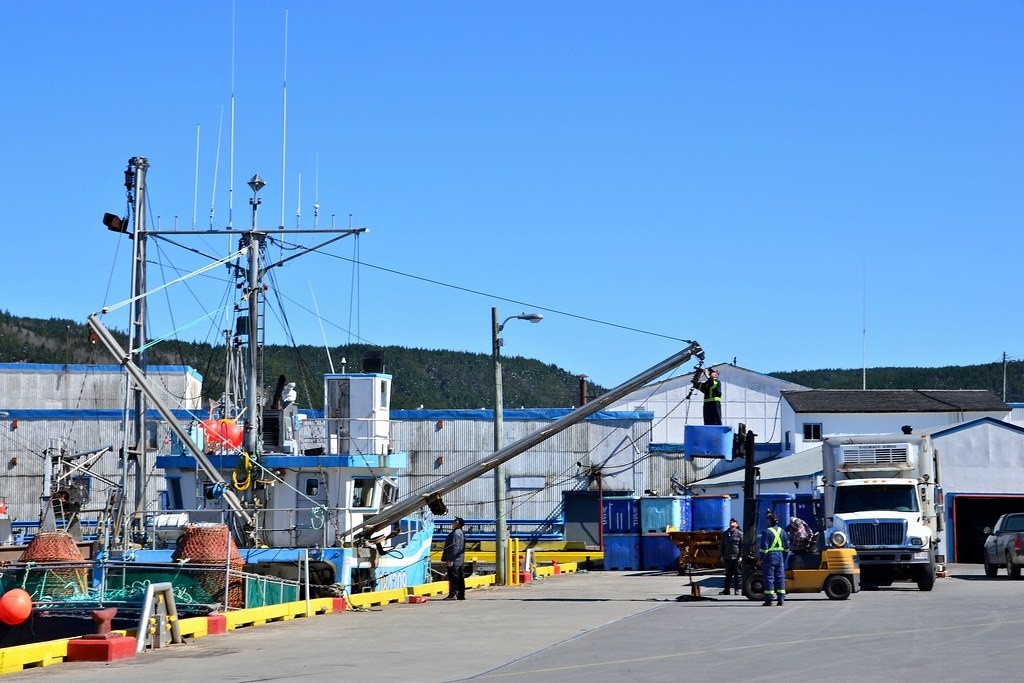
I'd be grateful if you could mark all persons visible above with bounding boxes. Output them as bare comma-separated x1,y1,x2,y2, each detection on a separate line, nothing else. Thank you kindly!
693,367,722,426
760,508,789,607
719,518,744,595
441,516,466,601
785,517,818,569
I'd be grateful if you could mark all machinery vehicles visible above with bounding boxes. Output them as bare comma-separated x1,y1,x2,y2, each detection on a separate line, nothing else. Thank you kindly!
690,422,862,600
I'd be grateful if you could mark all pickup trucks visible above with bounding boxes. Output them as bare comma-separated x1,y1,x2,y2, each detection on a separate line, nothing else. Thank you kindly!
982,512,1024,581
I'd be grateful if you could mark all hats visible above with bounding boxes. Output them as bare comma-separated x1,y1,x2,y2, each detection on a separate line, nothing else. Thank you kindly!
455,516,464,526
731,518,739,524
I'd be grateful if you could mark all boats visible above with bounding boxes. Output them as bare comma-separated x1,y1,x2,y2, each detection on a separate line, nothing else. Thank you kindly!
0,0,439,643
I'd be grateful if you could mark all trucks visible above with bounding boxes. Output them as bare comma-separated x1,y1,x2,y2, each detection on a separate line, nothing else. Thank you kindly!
812,425,946,593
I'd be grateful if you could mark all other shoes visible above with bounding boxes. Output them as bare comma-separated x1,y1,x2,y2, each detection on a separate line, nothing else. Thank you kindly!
776,602,784,606
735,589,740,595
457,595,466,600
762,602,772,606
719,589,730,595
442,595,457,600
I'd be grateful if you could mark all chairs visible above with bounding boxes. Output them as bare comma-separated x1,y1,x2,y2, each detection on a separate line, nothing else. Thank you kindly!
804,531,820,553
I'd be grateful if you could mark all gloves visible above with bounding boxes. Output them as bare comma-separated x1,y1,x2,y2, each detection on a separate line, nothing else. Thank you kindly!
721,556,724,562
737,557,742,565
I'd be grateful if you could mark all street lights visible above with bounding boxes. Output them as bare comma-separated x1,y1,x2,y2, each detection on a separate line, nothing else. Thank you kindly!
490,306,543,586
